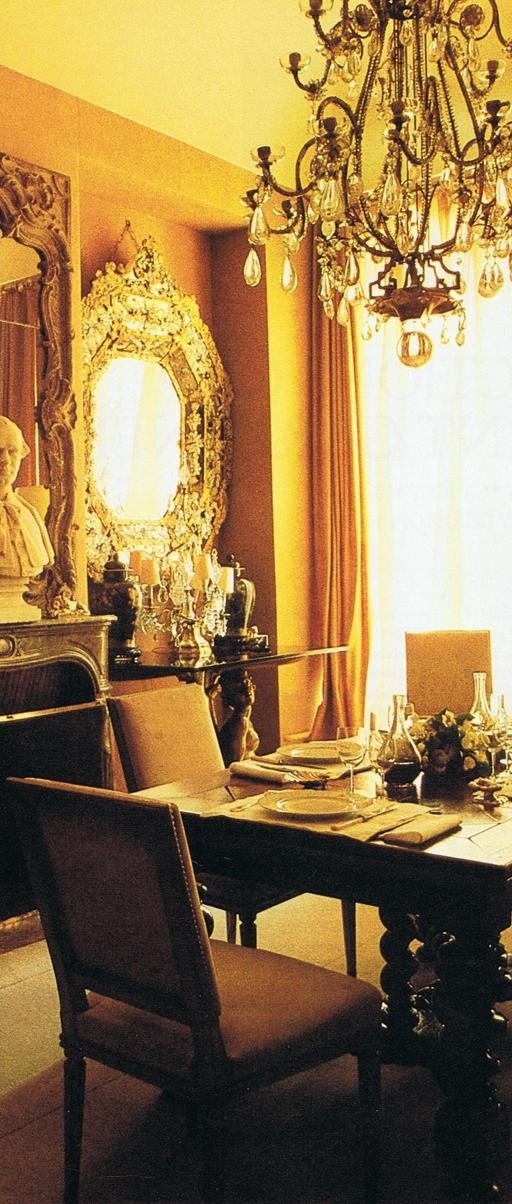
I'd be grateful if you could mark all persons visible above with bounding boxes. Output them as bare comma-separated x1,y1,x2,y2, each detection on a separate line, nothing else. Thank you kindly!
0,414,56,578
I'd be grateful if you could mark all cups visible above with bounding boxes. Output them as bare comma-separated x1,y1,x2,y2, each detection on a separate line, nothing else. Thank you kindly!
335,729,369,798
371,733,396,809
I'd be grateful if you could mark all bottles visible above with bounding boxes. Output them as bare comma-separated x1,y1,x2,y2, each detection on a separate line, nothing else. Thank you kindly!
377,695,421,789
468,670,494,729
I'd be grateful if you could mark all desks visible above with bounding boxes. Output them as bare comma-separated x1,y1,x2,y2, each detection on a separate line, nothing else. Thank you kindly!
101,640,353,766
0,615,121,955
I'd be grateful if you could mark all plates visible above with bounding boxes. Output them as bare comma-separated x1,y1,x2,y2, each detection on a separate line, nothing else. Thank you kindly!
273,738,367,760
256,787,369,817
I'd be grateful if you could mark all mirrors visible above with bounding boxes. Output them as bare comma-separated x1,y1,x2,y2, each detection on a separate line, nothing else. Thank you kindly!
0,170,78,619
81,230,233,646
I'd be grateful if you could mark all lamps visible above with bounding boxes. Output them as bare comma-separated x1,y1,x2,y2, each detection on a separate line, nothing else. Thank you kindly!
240,1,512,367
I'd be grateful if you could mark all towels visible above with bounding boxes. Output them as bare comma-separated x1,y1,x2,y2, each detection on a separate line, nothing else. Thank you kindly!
383,812,462,846
230,762,295,784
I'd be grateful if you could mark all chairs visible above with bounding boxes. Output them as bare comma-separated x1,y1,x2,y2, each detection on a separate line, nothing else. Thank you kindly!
104,683,356,977
3,775,386,1204
404,630,492,720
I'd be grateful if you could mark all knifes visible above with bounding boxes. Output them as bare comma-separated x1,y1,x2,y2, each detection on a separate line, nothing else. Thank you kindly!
326,806,419,830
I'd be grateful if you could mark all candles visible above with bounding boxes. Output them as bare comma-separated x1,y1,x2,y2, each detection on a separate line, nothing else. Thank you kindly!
192,552,212,579
138,558,161,584
128,550,145,575
218,567,234,593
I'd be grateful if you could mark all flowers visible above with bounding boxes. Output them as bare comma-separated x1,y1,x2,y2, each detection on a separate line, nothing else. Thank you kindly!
409,706,492,779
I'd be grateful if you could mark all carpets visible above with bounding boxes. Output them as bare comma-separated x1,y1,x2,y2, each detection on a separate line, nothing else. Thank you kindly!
0,893,511,1203
